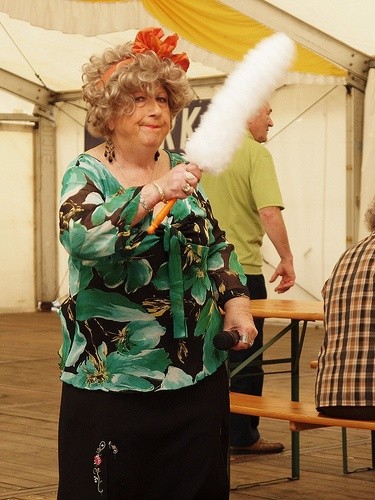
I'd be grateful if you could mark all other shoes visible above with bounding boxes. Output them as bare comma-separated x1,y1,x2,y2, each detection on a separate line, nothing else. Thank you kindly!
230,437,283,455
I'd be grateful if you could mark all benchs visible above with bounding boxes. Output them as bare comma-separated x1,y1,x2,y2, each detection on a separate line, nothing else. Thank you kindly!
230,393,375,431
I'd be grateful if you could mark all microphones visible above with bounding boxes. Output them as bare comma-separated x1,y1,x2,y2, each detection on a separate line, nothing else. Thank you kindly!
213,330,240,350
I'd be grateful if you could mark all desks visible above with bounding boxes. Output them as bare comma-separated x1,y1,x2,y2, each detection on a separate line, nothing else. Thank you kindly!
248,298,325,483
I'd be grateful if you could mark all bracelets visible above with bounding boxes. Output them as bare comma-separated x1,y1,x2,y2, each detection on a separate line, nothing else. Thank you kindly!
152,182,166,203
139,196,154,213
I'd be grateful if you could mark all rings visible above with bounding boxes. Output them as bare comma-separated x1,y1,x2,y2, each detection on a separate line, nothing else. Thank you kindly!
240,335,248,343
184,172,195,180
249,342,253,348
182,182,190,192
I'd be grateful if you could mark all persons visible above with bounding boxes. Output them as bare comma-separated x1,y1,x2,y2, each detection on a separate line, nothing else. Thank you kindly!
314,198,375,421
200,100,296,455
57,27,258,500
